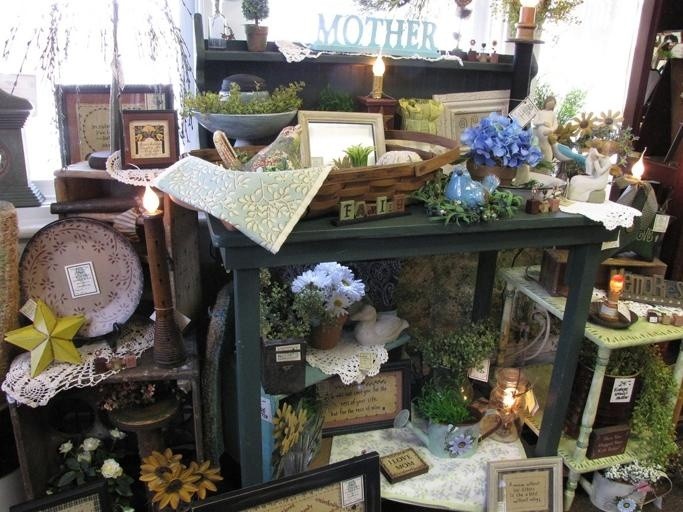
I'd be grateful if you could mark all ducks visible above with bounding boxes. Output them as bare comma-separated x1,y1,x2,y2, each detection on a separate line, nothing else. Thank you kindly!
349,305,410,346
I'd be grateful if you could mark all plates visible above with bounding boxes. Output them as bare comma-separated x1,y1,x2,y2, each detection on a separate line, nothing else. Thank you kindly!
589,302,638,328
17,216,147,341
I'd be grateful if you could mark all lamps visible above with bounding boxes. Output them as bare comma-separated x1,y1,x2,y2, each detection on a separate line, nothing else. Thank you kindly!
141,182,188,368
631,145,647,182
485,366,531,446
372,49,385,99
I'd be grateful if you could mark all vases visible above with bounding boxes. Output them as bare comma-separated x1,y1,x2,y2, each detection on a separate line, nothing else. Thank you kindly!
309,314,346,350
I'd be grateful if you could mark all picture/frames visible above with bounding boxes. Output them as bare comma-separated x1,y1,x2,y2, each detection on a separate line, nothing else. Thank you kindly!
55,84,174,167
433,89,510,140
122,110,179,169
191,451,381,511
322,359,411,438
9,480,110,512
298,110,386,167
486,456,563,512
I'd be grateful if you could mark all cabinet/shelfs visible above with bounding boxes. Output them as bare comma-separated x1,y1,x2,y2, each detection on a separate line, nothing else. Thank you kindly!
490,264,683,475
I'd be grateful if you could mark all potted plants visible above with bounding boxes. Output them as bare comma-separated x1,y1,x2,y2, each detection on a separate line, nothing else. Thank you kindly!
572,343,680,470
180,81,306,147
408,317,497,461
257,267,307,395
242,0,269,52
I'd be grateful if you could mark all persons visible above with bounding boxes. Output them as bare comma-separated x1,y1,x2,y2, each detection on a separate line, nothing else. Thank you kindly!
532,95,559,164
564,138,619,203
583,139,607,176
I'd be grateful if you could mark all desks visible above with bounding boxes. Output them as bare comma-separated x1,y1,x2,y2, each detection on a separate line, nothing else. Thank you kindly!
203,210,619,489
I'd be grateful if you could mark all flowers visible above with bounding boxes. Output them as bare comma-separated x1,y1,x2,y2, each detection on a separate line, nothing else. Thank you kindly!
57,428,223,511
270,404,306,480
290,262,367,318
